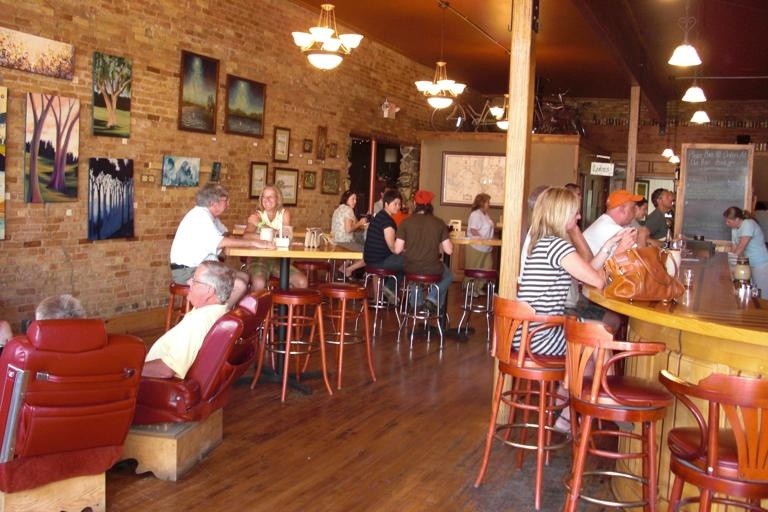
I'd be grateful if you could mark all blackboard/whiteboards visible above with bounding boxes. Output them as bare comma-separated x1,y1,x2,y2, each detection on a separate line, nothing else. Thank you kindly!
673,142,755,246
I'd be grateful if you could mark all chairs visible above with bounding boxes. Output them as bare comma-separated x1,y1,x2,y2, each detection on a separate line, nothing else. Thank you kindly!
109,290,276,486
562,317,672,511
0,317,146,512
472,294,600,511
655,369,768,511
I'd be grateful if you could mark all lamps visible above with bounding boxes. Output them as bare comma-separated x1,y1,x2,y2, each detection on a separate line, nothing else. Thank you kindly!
489,93,509,132
666,3,700,68
291,3,364,71
688,103,711,124
414,0,468,110
681,70,706,102
661,75,681,165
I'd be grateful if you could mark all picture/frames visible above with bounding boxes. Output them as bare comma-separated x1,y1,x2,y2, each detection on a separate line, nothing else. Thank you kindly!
248,161,268,199
273,166,299,207
271,124,290,164
176,48,220,134
303,124,340,195
439,151,505,209
223,73,266,138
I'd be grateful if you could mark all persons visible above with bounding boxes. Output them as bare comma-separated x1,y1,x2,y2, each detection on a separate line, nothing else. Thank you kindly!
241,185,309,292
722,206,768,301
141,259,237,382
0,295,88,353
168,182,249,311
511,183,676,438
331,185,455,314
463,192,495,297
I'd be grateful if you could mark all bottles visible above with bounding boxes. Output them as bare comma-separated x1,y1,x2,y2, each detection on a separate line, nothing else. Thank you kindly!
733,257,751,282
739,283,753,298
751,141,766,151
593,117,766,129
259,229,273,241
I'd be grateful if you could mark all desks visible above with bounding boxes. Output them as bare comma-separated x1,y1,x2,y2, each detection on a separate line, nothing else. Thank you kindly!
220,236,365,396
407,230,503,345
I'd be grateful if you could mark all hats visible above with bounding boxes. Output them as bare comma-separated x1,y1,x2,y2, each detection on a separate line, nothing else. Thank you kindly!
414,190,436,204
606,190,643,208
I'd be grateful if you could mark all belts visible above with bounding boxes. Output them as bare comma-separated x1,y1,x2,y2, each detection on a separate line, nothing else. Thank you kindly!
170,263,188,270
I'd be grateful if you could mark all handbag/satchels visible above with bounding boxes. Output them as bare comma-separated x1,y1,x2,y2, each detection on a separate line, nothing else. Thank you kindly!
604,247,685,301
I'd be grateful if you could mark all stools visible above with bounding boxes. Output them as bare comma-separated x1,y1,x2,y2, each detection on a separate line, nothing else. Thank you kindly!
268,275,302,386
302,282,378,389
165,282,193,332
354,267,400,337
457,269,495,352
249,288,334,401
291,259,335,336
396,271,444,352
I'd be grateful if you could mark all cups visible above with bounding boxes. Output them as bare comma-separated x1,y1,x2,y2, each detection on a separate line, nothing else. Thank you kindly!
669,240,678,250
278,226,294,243
677,239,685,250
446,224,453,233
682,268,694,289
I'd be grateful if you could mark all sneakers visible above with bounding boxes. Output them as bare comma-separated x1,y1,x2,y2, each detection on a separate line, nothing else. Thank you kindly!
335,272,485,312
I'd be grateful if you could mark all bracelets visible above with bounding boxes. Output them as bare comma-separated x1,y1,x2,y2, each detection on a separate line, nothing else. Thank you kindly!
257,221,265,230
